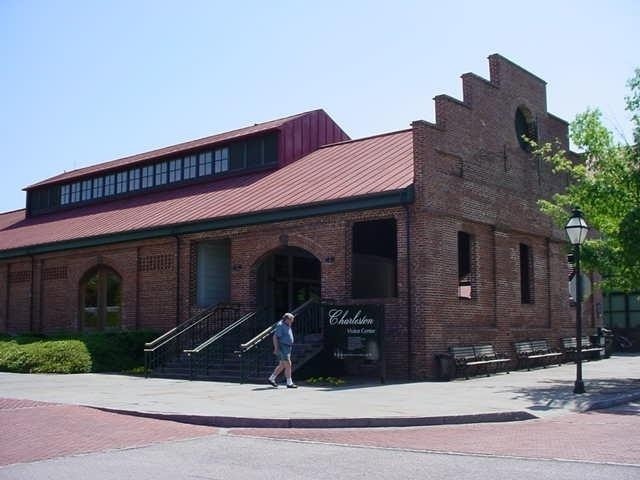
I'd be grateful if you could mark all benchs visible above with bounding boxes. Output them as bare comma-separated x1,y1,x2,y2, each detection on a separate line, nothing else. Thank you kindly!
434,341,513,381
559,335,607,361
510,338,564,371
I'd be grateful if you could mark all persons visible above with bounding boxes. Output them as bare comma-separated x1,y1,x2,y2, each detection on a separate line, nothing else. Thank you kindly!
267,312,298,388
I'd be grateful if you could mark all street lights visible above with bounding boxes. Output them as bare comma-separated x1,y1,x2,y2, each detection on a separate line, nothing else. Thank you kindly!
565,206,591,394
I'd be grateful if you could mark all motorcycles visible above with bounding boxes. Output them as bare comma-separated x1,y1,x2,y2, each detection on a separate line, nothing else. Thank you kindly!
606,322,633,357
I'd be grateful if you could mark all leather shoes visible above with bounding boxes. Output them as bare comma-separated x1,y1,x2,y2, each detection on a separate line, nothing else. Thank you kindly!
267,378,278,387
287,384,298,388
